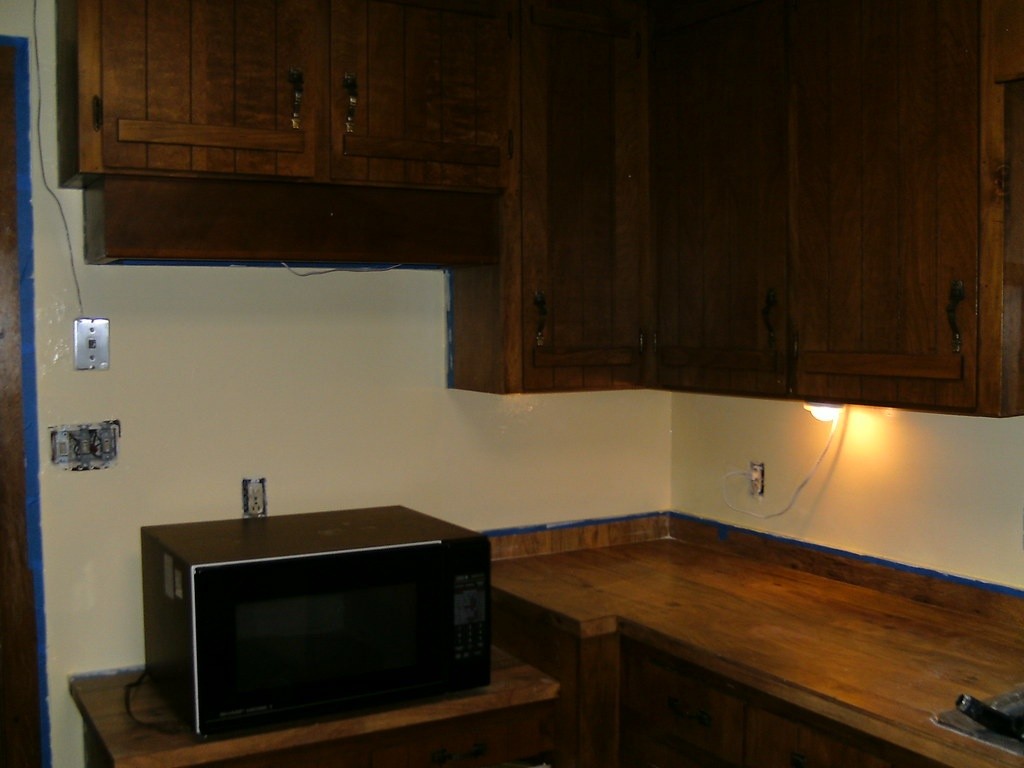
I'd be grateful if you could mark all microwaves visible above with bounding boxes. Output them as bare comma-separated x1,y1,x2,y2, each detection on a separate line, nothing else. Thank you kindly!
140,504,495,739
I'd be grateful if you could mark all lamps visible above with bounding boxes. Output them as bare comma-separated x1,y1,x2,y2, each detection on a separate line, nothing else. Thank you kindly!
800,402,846,423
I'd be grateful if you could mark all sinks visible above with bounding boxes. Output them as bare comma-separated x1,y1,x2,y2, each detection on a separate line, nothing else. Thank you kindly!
927,686,1024,760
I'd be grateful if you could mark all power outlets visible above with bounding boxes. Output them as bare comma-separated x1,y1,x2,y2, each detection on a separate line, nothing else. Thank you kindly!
750,459,766,497
242,476,267,519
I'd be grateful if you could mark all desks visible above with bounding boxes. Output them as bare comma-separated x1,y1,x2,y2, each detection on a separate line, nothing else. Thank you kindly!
66,642,554,768
55,1,514,199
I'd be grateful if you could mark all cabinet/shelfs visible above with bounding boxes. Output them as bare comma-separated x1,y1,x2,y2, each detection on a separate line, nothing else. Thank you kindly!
650,1,1024,417
447,0,644,393
617,620,921,767
